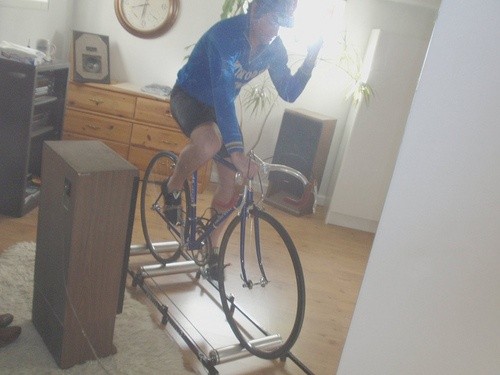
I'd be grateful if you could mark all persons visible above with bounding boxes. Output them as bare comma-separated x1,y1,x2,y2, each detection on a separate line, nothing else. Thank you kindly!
161,0,324,281
0,313,21,349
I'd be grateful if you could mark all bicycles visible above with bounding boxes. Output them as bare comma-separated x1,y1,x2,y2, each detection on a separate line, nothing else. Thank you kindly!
140,149,312,361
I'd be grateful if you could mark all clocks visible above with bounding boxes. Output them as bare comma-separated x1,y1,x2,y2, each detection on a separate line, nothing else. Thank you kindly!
114,0,180,39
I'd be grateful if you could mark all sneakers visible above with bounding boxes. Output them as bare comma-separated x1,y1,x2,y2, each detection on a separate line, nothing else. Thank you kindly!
161,178,183,226
207,254,220,280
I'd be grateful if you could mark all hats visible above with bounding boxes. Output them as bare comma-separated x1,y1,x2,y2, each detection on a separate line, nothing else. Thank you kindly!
260,0,297,27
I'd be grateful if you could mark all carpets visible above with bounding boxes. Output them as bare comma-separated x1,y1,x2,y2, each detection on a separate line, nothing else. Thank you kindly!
0,240,197,375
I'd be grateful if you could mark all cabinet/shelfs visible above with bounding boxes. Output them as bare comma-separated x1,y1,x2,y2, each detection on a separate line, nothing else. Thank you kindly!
0,53,71,219
61,77,215,194
29,139,140,370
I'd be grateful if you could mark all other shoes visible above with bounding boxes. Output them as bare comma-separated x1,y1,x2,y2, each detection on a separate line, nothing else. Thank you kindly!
0,325,21,347
0,313,14,327
